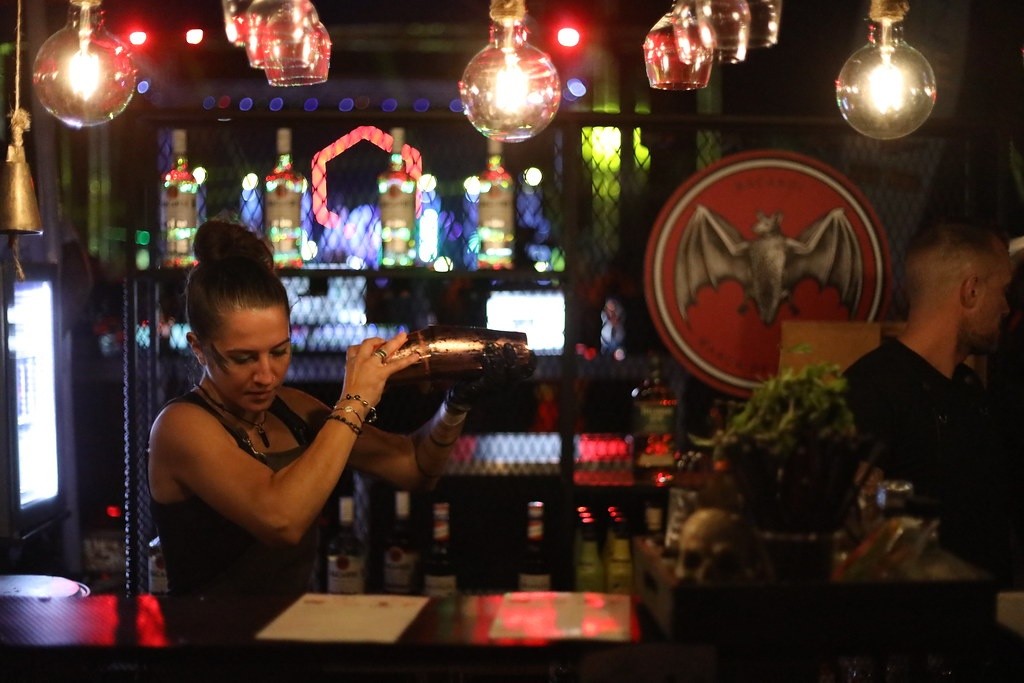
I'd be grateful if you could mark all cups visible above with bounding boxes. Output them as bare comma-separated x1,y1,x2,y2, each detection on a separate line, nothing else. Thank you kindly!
224,0,330,87
642,0,781,89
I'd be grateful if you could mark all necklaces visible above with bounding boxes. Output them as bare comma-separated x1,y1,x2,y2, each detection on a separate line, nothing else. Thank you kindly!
197,384,270,447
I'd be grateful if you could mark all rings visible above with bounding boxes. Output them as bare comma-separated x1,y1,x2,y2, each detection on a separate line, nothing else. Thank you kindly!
374,349,388,363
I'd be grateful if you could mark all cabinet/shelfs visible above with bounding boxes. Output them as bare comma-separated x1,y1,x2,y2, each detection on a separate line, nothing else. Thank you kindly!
77,97,959,596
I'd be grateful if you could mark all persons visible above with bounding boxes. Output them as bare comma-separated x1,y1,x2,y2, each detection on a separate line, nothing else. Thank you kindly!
585,294,642,361
777,221,1024,592
144,219,538,598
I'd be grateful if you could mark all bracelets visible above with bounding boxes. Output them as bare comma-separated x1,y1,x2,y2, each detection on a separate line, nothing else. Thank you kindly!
438,402,465,426
426,421,457,447
325,394,378,435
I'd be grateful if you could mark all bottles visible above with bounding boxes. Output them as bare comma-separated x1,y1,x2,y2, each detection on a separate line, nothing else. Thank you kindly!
158,129,198,268
376,128,417,268
478,138,514,270
631,354,675,477
265,129,304,267
147,533,170,597
327,487,667,598
